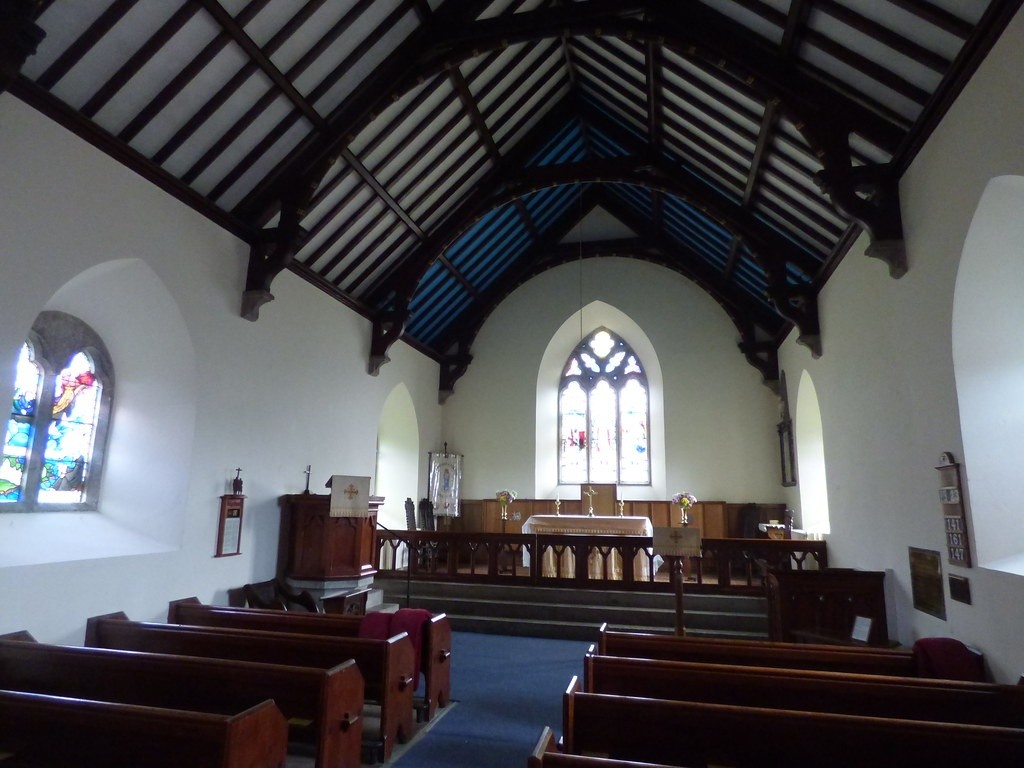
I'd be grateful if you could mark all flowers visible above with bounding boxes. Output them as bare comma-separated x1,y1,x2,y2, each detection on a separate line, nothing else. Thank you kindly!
496,490,517,505
672,492,697,510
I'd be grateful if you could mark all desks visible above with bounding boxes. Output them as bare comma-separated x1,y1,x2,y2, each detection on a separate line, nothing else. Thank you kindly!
522,514,654,581
790,622,899,649
766,568,887,642
701,538,828,594
653,527,700,583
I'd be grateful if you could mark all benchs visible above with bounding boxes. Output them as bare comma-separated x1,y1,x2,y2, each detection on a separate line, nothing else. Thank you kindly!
563,675,1024,766
584,642,1024,729
167,596,453,722
528,725,688,768
0,686,289,768
85,611,419,762
0,629,364,768
598,621,987,684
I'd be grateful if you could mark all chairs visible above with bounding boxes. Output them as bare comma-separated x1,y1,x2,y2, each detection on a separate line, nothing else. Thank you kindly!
744,508,794,586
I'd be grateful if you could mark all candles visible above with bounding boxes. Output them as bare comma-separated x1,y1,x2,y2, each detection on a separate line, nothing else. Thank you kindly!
556,492,559,501
583,486,599,507
621,488,623,503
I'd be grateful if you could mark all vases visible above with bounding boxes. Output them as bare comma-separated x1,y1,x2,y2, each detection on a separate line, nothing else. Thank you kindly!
501,506,507,519
681,508,688,523
